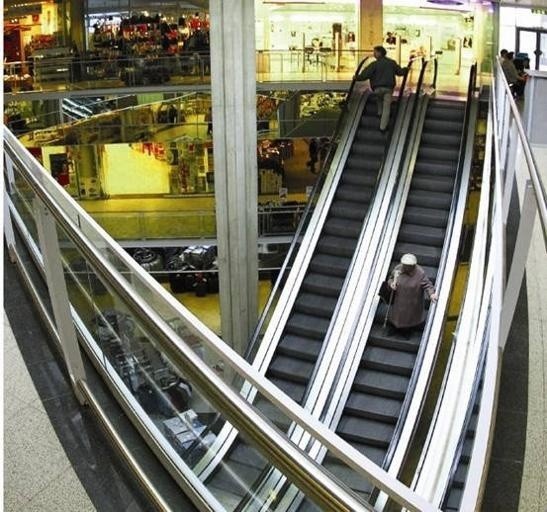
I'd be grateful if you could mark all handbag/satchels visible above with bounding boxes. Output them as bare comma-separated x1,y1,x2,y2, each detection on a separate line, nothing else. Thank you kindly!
378,281,395,305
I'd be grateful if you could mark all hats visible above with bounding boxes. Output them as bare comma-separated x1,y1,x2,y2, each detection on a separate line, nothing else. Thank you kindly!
401,253,417,265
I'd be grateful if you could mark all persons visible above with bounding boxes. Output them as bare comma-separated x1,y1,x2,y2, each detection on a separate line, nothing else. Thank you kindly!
385,253,438,341
353,46,410,133
208,107,212,135
386,32,396,44
316,137,331,169
307,137,319,174
499,49,529,100
